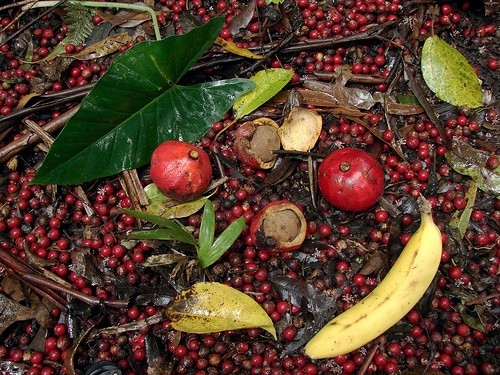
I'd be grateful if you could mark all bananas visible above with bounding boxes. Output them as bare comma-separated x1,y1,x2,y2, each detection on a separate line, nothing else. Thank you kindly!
303,191,443,360
